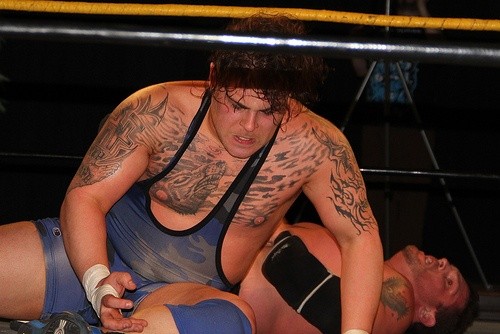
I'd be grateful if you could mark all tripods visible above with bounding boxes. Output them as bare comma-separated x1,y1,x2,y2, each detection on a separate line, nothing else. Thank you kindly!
295,52,493,290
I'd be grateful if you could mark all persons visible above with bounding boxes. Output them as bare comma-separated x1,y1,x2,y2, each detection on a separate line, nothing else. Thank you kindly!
230,217,480,334
351,1,436,124
0,14,384,334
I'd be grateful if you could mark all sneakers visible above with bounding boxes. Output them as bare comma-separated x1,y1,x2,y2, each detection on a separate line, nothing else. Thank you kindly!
10,310,94,334
